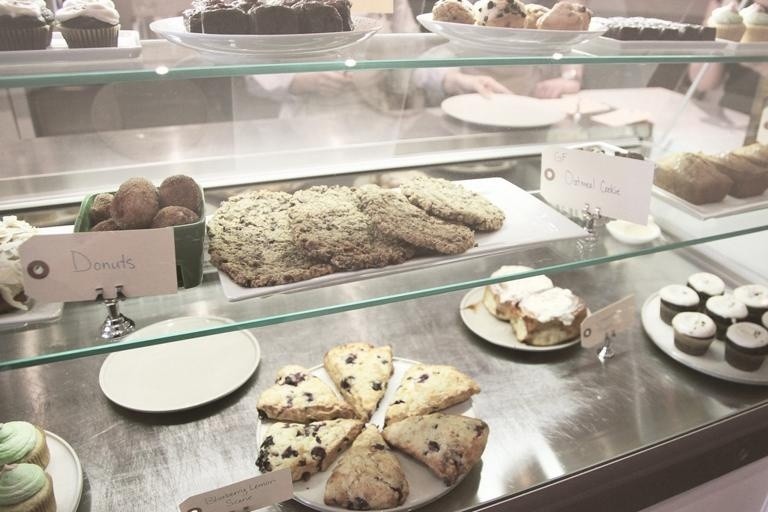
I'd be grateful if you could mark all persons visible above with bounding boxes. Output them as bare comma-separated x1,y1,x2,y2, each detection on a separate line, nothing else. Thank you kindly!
238,0,425,123
689,1,768,128
412,1,591,112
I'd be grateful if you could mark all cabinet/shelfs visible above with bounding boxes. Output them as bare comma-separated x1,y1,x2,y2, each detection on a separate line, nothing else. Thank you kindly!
0,23,768,510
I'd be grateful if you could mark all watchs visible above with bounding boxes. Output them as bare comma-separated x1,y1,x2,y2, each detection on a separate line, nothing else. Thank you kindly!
558,70,585,86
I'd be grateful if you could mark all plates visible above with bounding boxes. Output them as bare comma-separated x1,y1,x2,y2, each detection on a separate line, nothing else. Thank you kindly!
442,93,567,129
98,312,260,413
148,17,381,61
75,191,206,299
415,11,608,51
599,33,726,56
37,426,87,512
459,283,590,353
653,156,766,224
1,294,65,331
2,29,145,73
208,175,588,303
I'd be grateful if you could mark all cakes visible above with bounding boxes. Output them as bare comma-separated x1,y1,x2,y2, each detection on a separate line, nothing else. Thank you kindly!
482,264,587,346
0,421,57,512
0,0,120,51
660,272,768,372
0,215,45,314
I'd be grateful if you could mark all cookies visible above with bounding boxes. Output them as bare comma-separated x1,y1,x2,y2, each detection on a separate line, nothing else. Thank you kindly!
205,176,504,289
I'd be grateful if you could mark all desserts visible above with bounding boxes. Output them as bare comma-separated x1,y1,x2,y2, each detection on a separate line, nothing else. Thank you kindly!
431,1,594,44
593,12,717,41
705,1,768,44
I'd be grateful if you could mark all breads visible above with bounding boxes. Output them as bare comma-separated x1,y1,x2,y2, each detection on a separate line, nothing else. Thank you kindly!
573,143,768,206
182,0,356,35
87,176,202,229
